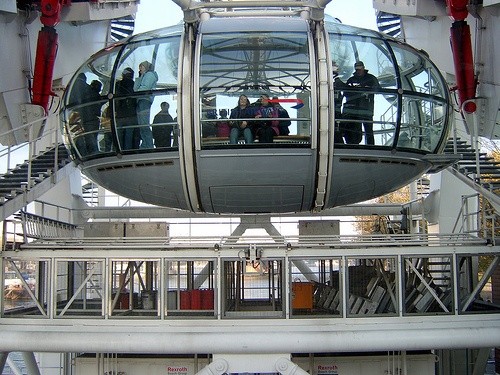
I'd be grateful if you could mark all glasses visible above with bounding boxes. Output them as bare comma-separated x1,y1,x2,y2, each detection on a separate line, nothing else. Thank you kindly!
240,98,247,100
355,67,364,71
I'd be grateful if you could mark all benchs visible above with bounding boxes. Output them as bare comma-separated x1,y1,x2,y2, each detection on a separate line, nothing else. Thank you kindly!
203,136,311,145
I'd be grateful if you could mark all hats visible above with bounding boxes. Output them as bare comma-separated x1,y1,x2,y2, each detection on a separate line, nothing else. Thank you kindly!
354,61,364,67
141,61,154,71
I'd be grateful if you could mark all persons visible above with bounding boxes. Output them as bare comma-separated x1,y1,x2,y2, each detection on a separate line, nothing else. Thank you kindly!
333,62,345,144
228,94,254,144
202,109,230,138
272,97,291,136
69,73,102,157
254,94,279,142
101,67,141,152
152,102,178,147
133,61,158,149
342,62,379,144
